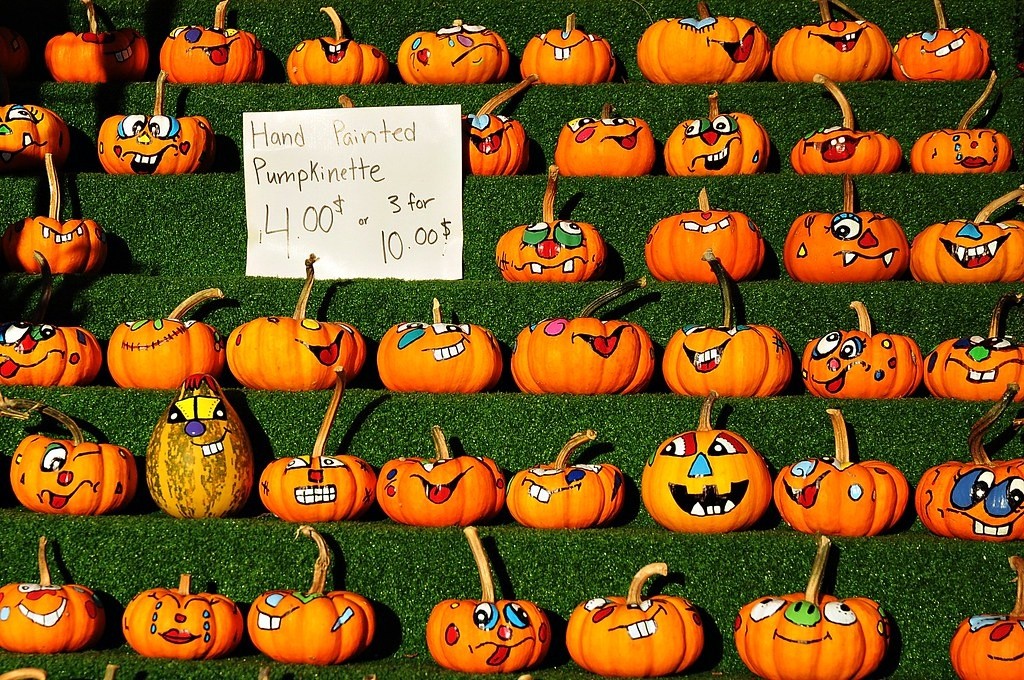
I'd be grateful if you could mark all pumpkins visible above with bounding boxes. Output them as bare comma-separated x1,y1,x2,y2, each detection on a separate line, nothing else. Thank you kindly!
0,0,1024,680
